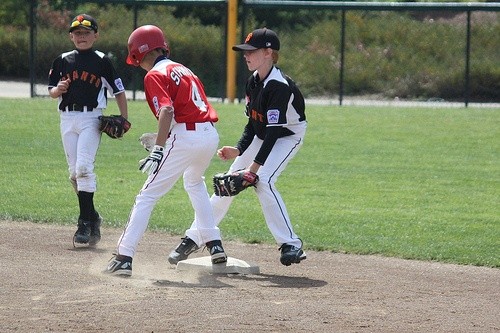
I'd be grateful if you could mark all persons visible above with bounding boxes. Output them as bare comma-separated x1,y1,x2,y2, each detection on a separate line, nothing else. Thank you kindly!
103,24,227,278
47,14,130,247
167,27,308,266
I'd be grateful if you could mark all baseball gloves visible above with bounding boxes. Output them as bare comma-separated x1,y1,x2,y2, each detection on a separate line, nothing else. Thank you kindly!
98,114,132,139
213,168,260,196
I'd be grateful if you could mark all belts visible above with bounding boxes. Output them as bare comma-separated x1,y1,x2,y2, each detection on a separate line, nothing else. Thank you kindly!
62,103,94,113
185,122,195,132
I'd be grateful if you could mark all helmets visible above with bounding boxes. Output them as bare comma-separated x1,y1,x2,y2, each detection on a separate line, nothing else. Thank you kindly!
127,25,170,65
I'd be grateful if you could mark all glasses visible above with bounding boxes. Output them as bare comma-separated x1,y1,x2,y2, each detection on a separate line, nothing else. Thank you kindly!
71,20,97,30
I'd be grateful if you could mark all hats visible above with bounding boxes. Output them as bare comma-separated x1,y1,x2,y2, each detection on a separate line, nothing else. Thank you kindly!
69,14,98,33
232,28,281,51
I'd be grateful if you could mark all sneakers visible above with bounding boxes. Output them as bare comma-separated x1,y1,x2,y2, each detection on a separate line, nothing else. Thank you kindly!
278,240,306,266
168,236,198,265
74,212,102,247
103,252,133,278
205,240,228,264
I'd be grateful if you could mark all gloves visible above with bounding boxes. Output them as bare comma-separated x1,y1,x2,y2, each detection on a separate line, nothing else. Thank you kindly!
138,145,165,176
